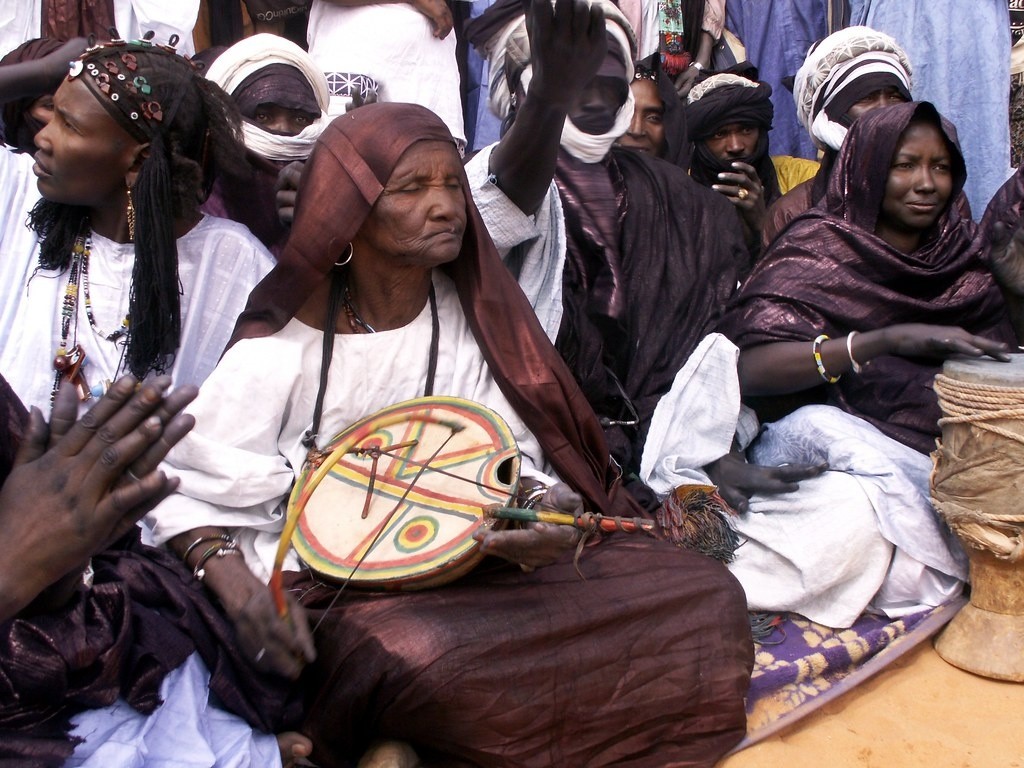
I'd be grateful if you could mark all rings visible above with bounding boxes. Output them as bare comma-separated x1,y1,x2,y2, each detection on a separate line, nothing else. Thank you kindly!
125,469,141,483
737,188,749,199
255,648,265,661
945,339,949,342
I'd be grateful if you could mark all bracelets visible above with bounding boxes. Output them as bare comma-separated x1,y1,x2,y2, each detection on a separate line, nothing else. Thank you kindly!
813,334,841,383
844,329,871,374
182,531,242,579
522,484,549,514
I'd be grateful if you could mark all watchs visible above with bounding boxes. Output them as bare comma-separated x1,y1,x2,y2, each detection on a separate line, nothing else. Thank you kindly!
689,61,704,70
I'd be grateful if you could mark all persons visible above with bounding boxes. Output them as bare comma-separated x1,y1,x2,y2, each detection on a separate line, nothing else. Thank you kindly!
733,100,1023,457
0,0,1010,265
458,1,896,627
137,102,752,768
0,38,280,423
0,369,318,768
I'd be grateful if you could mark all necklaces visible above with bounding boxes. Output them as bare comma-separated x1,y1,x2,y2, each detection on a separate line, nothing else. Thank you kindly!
47,216,134,414
340,284,375,332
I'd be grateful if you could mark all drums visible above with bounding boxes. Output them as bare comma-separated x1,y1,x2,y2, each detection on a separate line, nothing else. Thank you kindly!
932,353,1024,684
284,394,522,591
